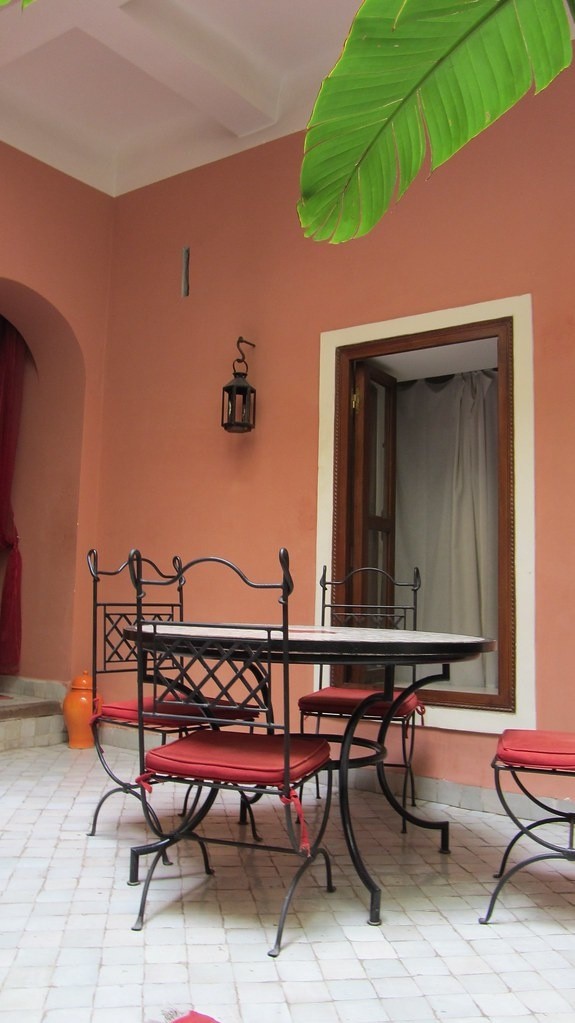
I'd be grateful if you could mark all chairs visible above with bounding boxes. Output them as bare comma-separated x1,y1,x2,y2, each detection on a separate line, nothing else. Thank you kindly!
297,566,425,833
478,729,575,924
85,549,262,874
128,547,338,956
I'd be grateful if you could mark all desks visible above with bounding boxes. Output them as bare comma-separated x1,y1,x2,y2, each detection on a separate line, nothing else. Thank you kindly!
121,623,498,927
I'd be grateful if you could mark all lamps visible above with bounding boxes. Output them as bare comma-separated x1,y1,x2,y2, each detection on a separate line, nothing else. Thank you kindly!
222,337,258,434
